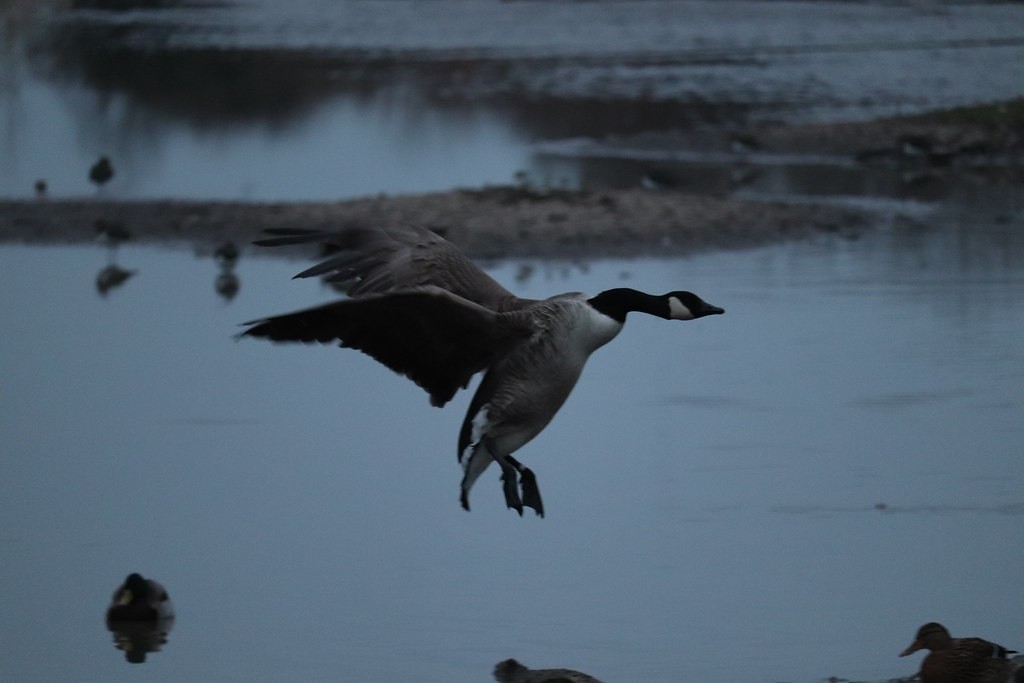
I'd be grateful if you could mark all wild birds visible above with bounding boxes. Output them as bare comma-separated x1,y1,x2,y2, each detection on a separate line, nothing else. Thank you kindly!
900,136,932,158
91,220,130,250
639,171,670,192
97,249,136,299
728,132,762,154
216,268,240,303
106,574,178,629
901,623,1024,683
236,221,724,519
34,180,47,193
493,658,600,683
213,242,236,271
89,158,112,185
